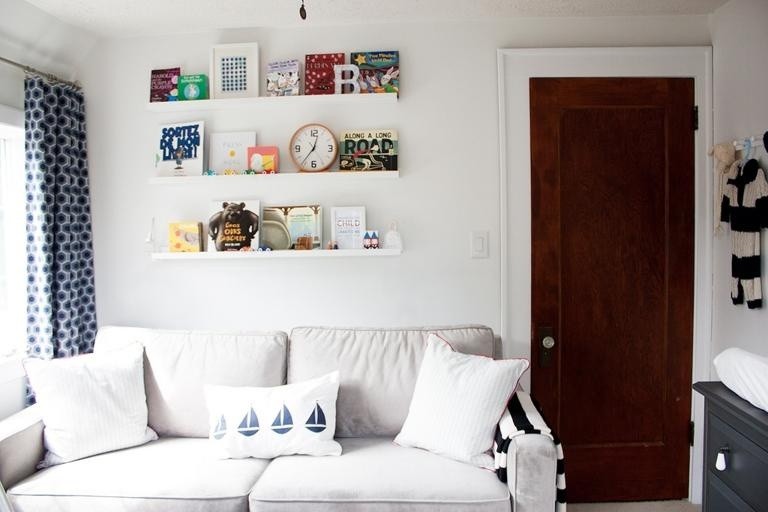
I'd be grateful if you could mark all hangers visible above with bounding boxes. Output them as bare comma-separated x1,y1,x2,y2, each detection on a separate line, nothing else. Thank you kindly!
725,137,765,186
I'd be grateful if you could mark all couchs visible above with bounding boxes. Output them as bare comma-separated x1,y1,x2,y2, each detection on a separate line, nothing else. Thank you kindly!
1,323,559,511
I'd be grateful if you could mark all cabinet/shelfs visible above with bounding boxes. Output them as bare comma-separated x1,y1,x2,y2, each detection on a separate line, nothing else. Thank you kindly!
152,94,401,259
688,375,768,512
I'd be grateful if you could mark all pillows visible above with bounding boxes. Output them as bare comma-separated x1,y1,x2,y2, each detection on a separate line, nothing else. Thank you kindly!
393,334,530,472
22,339,159,470
201,370,343,461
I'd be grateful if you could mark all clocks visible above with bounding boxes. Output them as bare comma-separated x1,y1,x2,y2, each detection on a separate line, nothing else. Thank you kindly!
289,122,339,172
